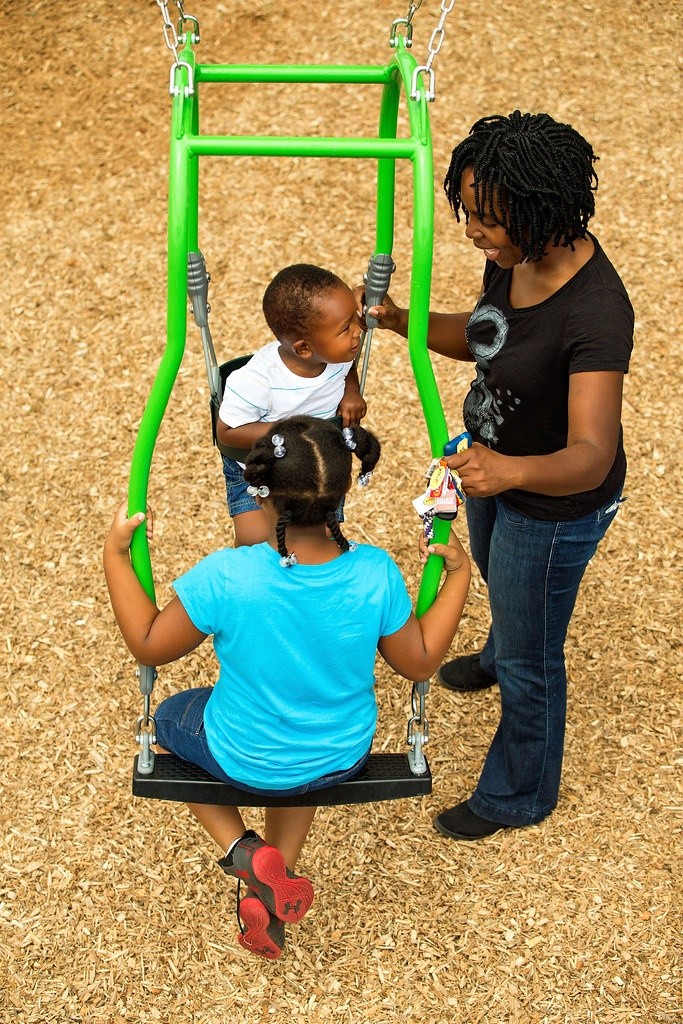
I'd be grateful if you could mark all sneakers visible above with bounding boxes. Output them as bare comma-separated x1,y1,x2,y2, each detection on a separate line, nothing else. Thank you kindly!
218,830,314,924
237,890,285,960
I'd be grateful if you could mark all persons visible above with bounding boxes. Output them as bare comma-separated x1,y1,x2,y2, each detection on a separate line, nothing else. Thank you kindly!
215,263,368,549
103,416,470,958
352,108,636,843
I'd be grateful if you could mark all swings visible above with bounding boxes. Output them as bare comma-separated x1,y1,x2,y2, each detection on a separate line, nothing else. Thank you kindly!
128,0,454,808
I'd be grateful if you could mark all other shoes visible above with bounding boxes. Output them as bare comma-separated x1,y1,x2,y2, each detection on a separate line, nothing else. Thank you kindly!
437,653,498,690
434,801,511,840
